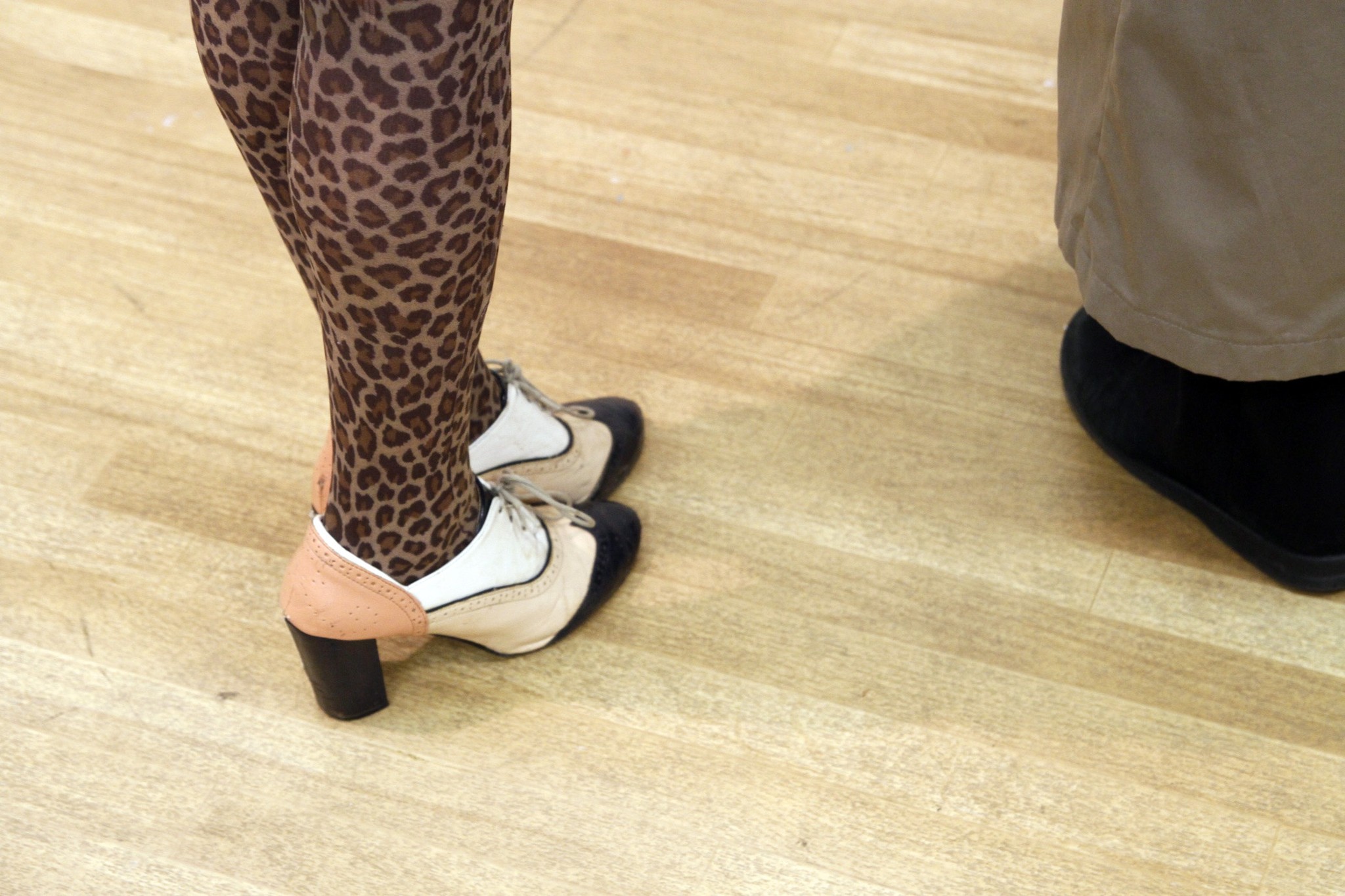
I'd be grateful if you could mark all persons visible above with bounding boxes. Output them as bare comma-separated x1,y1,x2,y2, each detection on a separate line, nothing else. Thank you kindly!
186,0,647,721
1053,0,1345,600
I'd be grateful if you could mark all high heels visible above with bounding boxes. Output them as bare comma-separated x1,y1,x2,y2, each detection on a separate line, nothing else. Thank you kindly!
310,359,644,517
277,472,642,722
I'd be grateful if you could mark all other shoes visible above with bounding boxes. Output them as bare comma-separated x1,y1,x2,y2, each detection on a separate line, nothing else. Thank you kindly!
1060,305,1345,599
